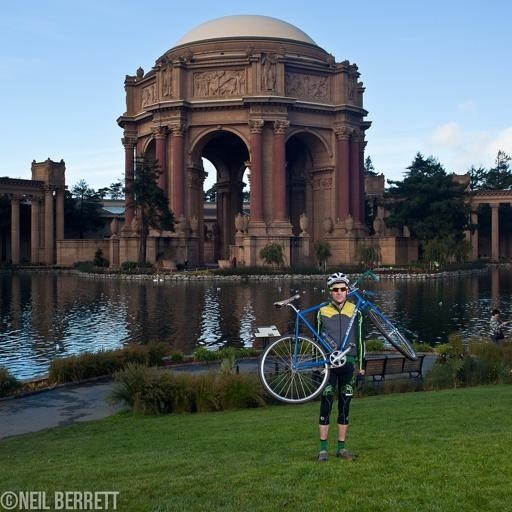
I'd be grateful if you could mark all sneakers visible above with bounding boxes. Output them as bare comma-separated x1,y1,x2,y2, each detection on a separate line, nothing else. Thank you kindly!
335,449,355,460
318,449,329,462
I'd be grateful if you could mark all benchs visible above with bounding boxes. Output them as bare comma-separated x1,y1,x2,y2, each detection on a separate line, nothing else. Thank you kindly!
364,355,425,385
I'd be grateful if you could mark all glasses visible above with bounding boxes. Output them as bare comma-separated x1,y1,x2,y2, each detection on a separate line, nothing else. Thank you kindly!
329,286,348,292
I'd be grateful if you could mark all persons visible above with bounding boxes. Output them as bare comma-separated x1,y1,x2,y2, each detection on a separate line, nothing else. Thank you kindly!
489,308,506,346
371,261,377,269
184,258,189,271
360,260,368,274
319,261,322,271
232,255,237,268
311,271,368,463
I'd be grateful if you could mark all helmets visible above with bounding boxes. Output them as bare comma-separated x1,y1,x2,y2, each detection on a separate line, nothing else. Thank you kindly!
326,271,350,288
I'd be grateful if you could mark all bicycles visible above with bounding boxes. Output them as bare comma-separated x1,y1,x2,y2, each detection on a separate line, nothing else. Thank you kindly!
258,271,417,404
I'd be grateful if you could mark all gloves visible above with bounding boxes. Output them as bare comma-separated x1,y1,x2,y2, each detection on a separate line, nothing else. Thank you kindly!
355,372,364,391
311,369,323,389
